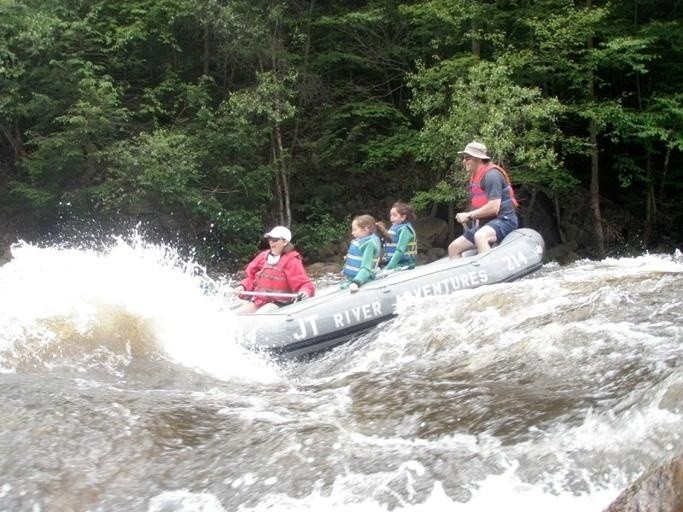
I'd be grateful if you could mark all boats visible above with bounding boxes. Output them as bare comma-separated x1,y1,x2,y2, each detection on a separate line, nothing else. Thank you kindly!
230,226,548,370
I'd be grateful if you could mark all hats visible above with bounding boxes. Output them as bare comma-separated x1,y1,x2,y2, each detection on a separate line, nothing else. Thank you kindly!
457,142,491,160
264,226,292,241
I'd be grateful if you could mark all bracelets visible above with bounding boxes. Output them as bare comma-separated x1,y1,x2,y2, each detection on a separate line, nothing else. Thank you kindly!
466,212,472,220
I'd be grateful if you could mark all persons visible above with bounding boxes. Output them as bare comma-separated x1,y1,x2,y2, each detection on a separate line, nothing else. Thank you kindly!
444,142,519,260
222,225,315,316
377,201,419,277
337,214,393,294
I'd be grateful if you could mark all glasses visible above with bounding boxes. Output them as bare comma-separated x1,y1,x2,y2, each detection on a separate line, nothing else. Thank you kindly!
462,156,470,161
